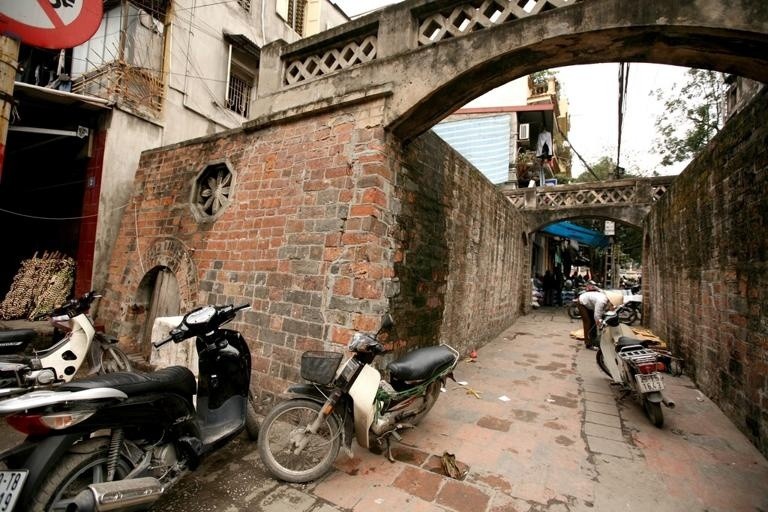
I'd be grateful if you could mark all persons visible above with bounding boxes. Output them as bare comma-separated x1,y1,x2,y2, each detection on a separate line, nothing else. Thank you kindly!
542,269,555,307
576,290,624,351
552,266,566,307
534,273,543,289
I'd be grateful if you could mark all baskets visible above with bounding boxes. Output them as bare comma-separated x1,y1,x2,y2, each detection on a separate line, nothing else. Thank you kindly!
300,346,344,386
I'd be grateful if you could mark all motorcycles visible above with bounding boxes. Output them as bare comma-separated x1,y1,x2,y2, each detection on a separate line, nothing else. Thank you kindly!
256,309,461,480
0,290,133,399
567,272,645,326
594,300,677,427
0,302,259,511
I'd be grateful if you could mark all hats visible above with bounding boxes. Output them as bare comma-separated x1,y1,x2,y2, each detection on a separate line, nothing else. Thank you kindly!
603,290,623,309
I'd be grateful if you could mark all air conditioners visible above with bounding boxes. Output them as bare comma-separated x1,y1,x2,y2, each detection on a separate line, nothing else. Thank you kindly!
519,123,532,148
